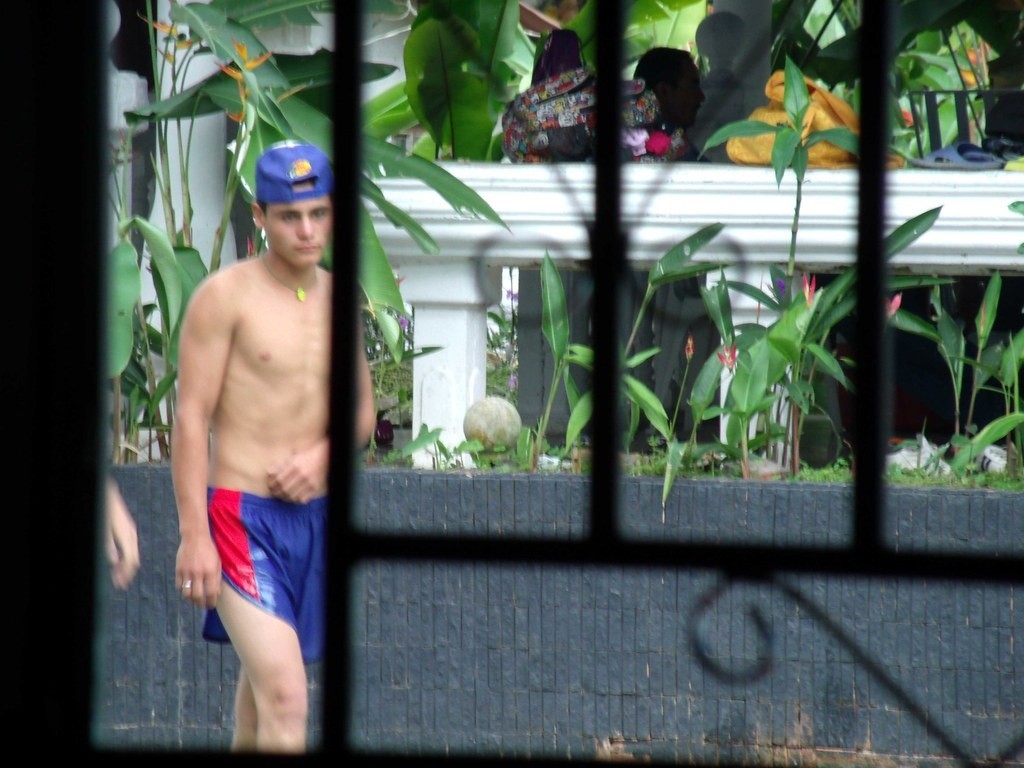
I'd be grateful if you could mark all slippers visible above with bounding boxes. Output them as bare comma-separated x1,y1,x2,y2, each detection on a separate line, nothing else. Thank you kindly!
911,140,1003,170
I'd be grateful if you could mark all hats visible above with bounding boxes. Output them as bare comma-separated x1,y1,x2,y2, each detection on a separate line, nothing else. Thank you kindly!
254,139,334,204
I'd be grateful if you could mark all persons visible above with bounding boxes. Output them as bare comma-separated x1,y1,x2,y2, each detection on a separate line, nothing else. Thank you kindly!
169,140,375,754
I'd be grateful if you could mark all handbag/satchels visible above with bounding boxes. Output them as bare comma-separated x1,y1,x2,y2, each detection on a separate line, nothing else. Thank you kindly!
501,67,686,165
725,71,905,169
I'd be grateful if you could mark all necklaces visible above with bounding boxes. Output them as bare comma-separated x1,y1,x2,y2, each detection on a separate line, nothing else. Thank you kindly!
260,255,315,302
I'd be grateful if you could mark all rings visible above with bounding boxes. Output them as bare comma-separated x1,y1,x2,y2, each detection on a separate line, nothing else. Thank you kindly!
181,580,192,588
